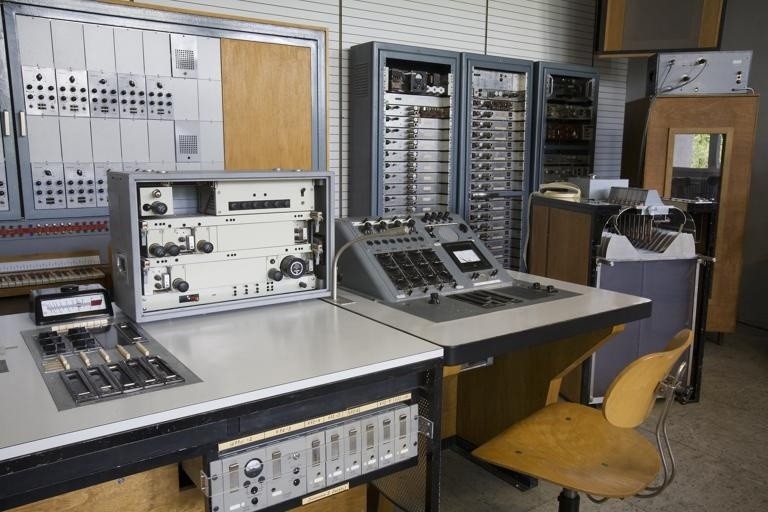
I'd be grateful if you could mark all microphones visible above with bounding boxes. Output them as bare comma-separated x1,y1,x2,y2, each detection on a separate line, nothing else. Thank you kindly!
324,225,410,306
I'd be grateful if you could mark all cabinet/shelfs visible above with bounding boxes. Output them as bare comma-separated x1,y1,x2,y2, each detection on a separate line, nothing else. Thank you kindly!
623,93,760,345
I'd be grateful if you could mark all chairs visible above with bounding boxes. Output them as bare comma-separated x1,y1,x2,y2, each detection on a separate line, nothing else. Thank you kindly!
471,329,697,512
